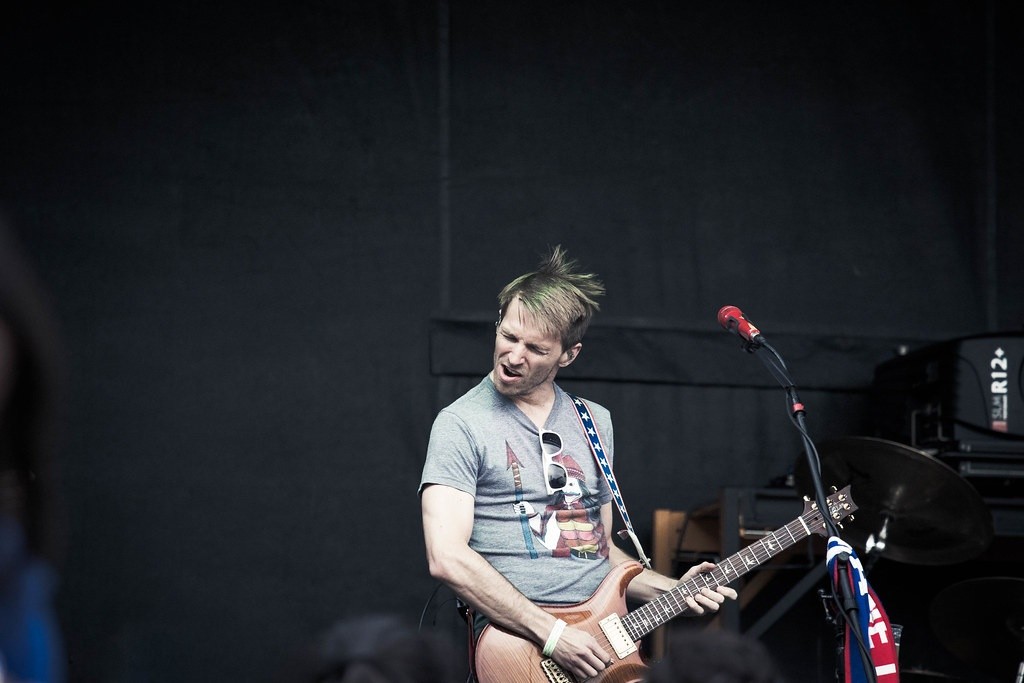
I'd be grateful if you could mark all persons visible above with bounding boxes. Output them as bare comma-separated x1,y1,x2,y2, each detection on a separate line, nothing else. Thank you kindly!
418,245,739,683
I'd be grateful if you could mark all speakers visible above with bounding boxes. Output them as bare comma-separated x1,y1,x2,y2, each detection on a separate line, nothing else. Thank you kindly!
646,484,842,683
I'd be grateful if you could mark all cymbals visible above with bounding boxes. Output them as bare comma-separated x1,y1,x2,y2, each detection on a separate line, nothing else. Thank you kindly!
789,427,998,572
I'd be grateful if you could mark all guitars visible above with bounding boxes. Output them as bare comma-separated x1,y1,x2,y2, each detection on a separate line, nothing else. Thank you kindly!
468,475,871,683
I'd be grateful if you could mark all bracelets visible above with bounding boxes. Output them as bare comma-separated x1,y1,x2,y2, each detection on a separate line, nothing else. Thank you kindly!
541,618,567,656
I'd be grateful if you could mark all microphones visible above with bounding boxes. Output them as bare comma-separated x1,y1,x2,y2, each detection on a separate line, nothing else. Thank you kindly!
717,305,766,346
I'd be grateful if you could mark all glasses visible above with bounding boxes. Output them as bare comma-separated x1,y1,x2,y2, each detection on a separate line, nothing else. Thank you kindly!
539,428,568,496
